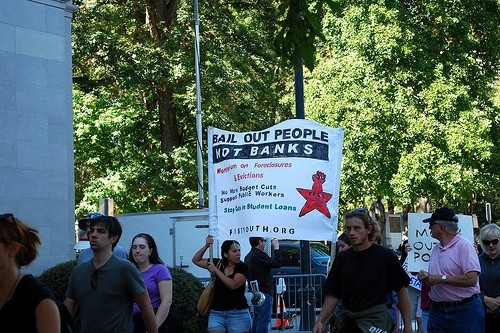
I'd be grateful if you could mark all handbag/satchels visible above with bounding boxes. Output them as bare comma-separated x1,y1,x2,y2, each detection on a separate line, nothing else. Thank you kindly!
197,260,223,315
421,290,431,309
57,302,74,333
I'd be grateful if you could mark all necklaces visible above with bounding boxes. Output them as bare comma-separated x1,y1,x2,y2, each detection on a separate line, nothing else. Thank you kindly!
5,270,21,305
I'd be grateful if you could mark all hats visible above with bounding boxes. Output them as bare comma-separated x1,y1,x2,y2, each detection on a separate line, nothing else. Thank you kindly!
79,213,104,231
423,208,458,223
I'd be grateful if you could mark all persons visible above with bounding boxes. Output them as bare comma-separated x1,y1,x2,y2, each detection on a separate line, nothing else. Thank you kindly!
63,217,158,333
336,223,483,333
244,237,282,333
417,208,484,333
191,234,252,333
477,224,500,333
312,209,413,333
128,233,172,329
0,212,61,333
76,213,128,268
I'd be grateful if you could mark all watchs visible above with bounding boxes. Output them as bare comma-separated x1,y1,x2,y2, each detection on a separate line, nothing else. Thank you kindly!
441,275,447,283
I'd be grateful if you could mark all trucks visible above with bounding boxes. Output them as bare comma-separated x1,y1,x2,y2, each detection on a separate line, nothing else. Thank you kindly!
114,207,332,308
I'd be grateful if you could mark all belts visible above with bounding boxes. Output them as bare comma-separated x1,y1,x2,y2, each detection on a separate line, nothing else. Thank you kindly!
430,292,480,307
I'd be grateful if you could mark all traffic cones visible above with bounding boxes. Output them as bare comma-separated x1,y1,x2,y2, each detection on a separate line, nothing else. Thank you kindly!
271,293,294,330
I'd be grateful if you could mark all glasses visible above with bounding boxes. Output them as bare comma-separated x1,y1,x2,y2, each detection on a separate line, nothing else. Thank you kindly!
358,208,371,223
481,237,499,246
91,270,97,291
258,239,266,243
0,214,20,243
430,221,446,227
